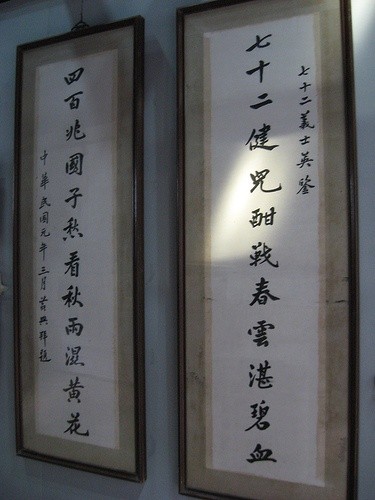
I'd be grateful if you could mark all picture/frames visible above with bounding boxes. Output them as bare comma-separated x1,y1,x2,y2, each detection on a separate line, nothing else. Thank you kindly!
11,15,148,485
175,0,358,500
